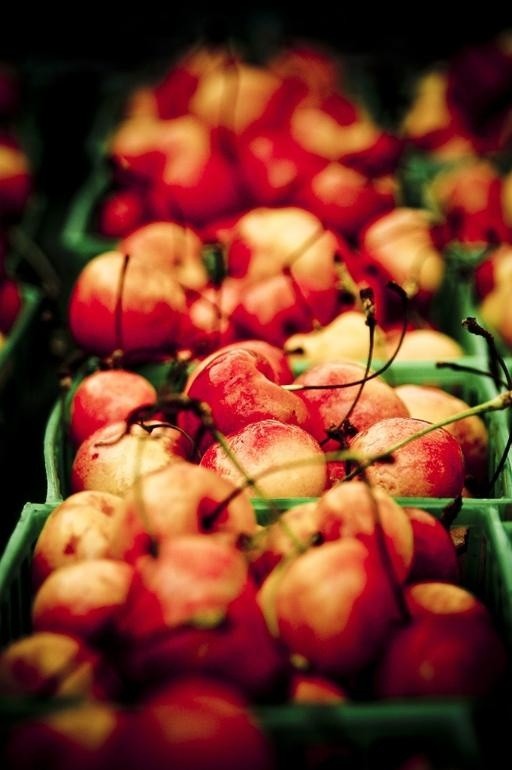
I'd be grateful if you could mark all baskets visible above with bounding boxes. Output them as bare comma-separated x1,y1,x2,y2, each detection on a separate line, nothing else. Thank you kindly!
0,94,512,770
2,41,510,769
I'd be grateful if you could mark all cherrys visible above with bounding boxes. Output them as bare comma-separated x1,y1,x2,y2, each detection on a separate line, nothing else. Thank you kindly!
0,0,512,770
